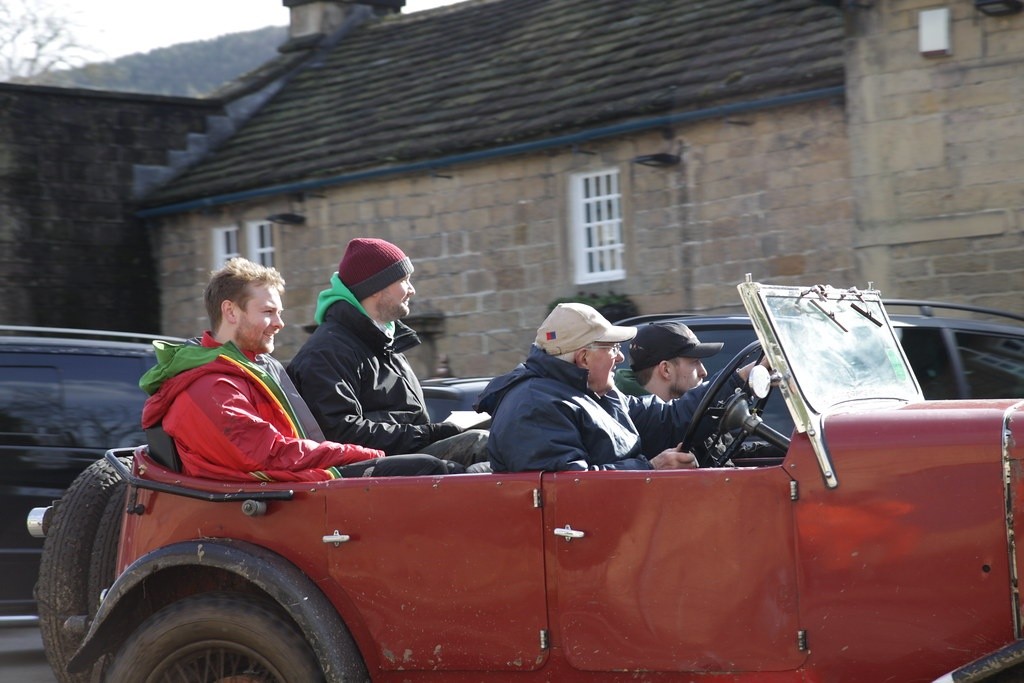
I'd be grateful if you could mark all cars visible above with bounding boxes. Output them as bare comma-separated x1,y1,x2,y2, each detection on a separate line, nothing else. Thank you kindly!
614,315,1023,445
421,376,497,420
22,274,1024,683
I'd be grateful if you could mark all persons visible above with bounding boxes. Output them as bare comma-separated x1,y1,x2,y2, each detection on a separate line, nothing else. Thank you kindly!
612,320,769,468
469,303,772,473
283,237,491,466
138,256,466,483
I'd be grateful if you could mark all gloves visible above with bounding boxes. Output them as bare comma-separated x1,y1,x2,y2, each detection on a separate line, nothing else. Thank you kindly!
425,422,468,444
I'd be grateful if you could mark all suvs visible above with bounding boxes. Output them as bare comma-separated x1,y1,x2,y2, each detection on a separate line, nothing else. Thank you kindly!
0,324,186,627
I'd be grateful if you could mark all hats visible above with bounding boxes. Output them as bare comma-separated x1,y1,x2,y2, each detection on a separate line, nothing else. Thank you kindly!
629,322,725,372
338,238,414,303
536,302,638,356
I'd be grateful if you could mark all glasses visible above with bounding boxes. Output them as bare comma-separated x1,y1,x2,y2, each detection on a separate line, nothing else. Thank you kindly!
585,342,622,360
